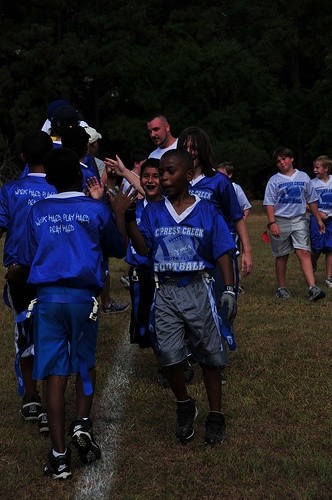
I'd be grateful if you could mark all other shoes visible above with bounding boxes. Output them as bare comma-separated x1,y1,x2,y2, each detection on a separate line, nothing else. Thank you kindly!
325,277,332,288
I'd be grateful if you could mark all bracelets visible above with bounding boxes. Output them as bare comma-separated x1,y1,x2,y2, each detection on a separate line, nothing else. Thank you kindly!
267,222,276,228
224,285,236,293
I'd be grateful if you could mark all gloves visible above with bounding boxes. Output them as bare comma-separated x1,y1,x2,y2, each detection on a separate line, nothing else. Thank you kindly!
220,285,238,322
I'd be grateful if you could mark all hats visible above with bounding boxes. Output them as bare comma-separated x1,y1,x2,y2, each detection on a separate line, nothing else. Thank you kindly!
47,100,80,130
84,127,102,144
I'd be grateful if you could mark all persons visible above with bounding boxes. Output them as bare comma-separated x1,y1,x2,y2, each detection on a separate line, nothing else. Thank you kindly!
125,149,236,444
17,147,128,480
306,156,332,287
263,146,325,302
0,98,253,434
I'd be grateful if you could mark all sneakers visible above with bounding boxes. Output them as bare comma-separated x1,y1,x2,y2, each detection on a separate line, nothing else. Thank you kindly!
276,287,290,298
174,395,199,444
67,417,101,465
19,396,42,421
308,285,326,302
43,447,73,481
36,412,49,434
204,411,226,445
100,299,130,315
